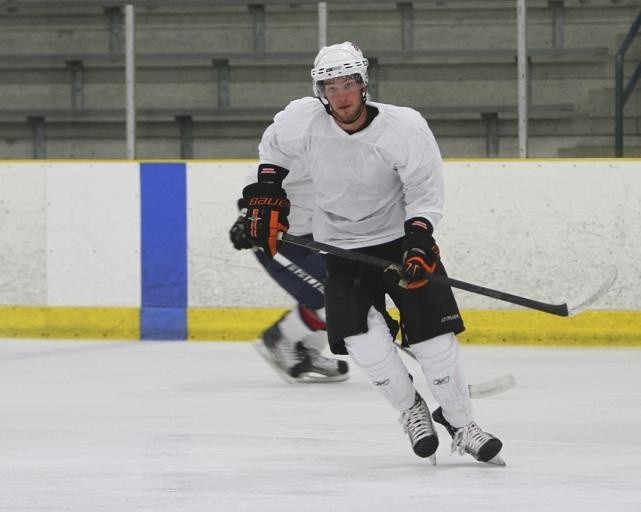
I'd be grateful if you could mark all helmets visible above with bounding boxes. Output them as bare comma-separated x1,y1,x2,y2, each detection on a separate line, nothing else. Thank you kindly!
310,41,370,105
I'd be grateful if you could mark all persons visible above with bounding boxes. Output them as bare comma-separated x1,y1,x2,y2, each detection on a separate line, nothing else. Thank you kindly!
252,231,348,388
229,39,502,463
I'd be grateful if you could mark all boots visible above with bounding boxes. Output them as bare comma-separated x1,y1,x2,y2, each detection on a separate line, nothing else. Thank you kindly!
263,311,349,377
396,372,439,458
432,406,503,462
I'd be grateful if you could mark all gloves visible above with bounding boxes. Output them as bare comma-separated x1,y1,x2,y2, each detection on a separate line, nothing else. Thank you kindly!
400,218,440,290
230,183,292,259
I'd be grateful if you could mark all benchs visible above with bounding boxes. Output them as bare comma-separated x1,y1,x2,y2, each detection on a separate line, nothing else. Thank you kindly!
0,1,640,158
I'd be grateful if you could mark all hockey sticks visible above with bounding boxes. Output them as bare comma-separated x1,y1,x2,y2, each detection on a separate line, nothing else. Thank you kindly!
266,255,515,399
275,230,618,318
